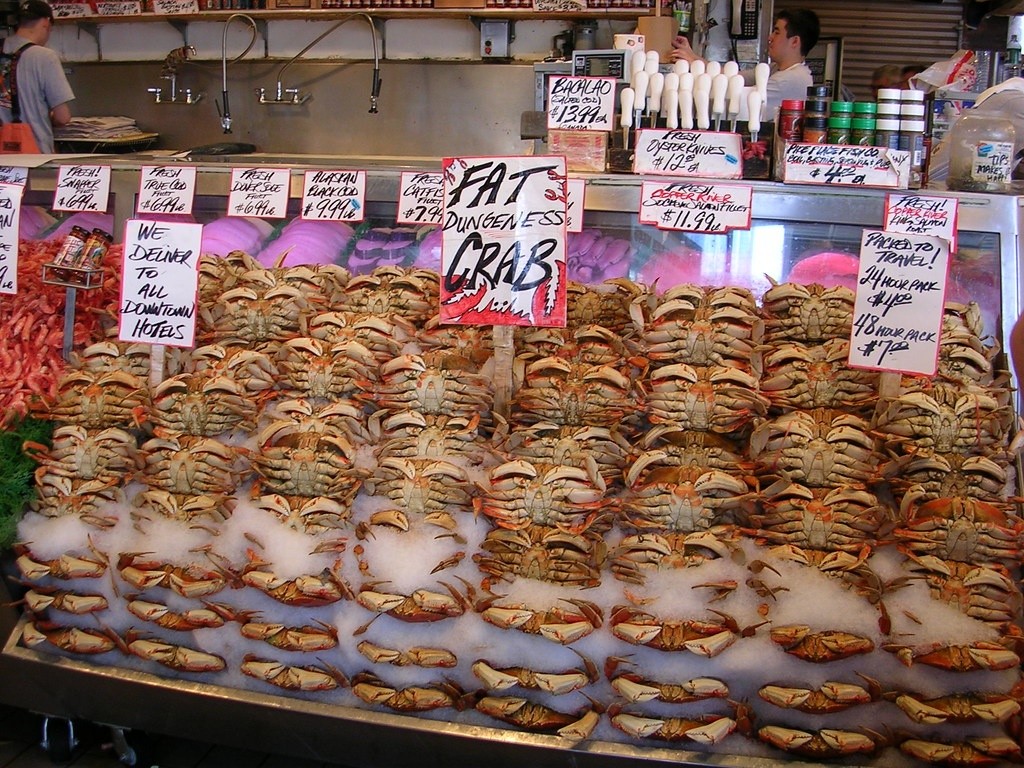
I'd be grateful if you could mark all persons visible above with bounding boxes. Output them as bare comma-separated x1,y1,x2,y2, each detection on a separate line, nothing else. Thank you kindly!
0,0,76,154
667,9,821,125
869,65,932,102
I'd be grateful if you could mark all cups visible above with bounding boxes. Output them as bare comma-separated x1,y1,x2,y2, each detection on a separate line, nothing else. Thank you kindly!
1003,63,1024,80
673,10,691,32
974,50,991,92
993,52,1009,86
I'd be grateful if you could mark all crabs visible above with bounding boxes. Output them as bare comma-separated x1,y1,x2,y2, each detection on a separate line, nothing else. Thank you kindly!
0,250,1024,768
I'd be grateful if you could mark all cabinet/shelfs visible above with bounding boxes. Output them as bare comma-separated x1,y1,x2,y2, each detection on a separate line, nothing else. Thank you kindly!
45,0,695,71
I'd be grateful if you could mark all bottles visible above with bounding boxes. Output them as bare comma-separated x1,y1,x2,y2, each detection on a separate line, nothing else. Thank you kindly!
1007,35,1022,65
947,109,1014,192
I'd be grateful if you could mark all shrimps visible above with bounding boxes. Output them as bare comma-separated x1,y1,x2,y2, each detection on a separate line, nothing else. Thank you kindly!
0,232,126,433
